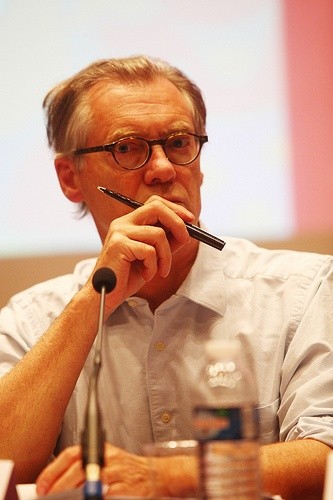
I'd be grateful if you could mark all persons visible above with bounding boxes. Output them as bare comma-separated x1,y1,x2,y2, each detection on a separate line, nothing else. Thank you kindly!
0,56,333,500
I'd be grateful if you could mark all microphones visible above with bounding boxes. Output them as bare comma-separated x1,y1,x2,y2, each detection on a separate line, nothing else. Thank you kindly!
79,267,117,500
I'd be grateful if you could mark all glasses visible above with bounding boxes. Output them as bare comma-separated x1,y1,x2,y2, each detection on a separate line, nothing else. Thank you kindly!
72,132,208,170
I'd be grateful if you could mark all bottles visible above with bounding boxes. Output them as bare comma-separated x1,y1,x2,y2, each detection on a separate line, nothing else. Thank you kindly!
192,338,261,500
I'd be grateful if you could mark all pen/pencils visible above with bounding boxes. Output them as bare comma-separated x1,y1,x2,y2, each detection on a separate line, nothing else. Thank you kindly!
96,185,227,252
